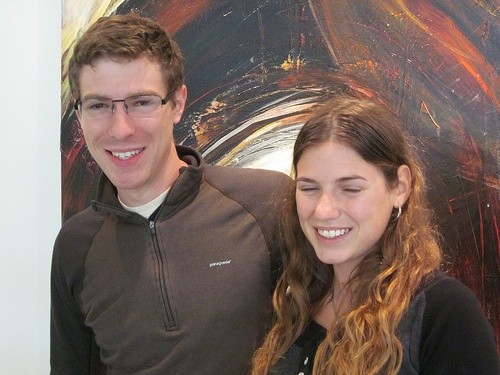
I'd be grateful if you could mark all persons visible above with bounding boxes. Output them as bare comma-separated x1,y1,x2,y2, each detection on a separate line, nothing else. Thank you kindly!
248,96,500,375
47,12,296,375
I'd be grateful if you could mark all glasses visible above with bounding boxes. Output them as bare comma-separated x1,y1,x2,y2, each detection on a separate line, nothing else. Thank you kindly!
75,87,177,120
